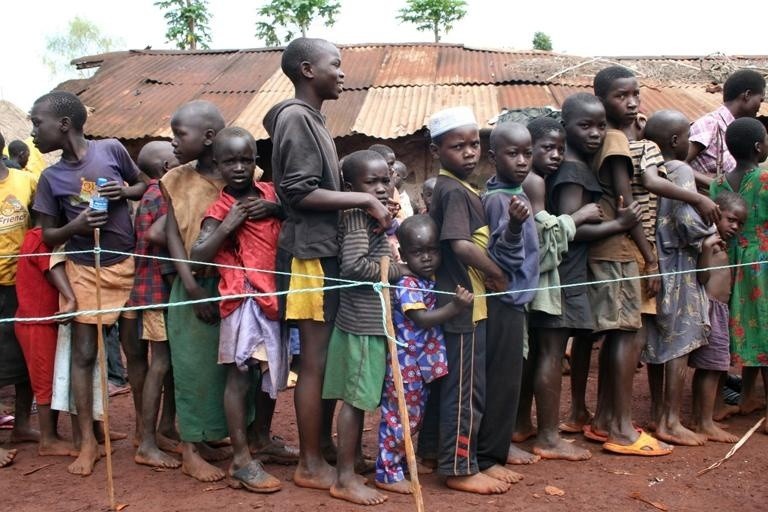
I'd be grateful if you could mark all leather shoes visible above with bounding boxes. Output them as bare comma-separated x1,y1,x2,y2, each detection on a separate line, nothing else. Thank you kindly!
426,105,480,142
250,441,302,465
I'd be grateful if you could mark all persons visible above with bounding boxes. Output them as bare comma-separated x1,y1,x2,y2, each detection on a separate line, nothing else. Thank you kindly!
420,64,768,494
0,37,477,504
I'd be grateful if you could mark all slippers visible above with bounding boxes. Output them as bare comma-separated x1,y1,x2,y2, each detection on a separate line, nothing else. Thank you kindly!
1,414,16,429
602,426,675,457
557,404,645,442
107,382,131,397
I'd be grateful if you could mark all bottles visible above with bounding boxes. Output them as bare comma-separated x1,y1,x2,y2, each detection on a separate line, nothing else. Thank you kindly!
86,177,111,215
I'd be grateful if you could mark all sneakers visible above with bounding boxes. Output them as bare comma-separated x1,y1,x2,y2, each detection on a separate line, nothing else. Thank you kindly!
227,457,282,494
